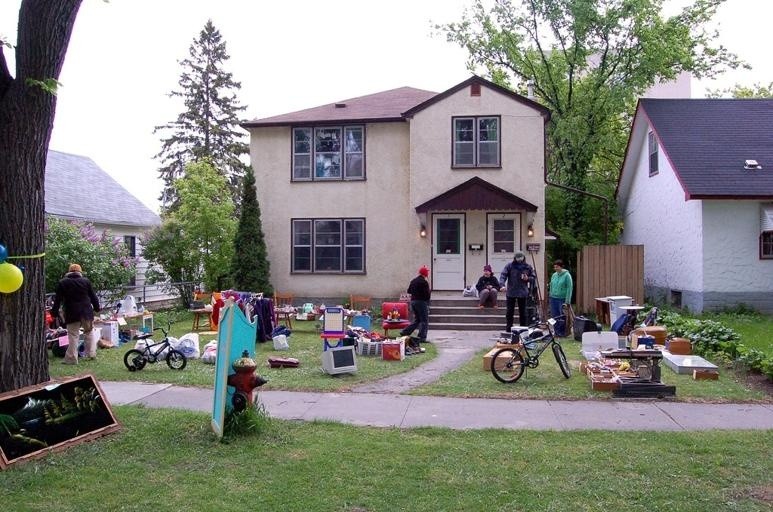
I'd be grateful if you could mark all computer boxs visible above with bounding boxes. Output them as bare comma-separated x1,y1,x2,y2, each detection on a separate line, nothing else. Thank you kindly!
100,321,120,346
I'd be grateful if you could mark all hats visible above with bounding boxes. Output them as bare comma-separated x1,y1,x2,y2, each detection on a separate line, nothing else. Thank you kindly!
515,251,524,261
484,265,491,272
419,265,429,277
69,264,82,271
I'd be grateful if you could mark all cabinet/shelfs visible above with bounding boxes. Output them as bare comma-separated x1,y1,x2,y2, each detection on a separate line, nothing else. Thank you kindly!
594,296,634,328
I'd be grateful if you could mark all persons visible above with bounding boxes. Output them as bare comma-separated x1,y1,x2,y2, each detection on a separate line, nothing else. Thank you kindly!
400,265,431,343
499,252,536,332
51,263,100,365
476,265,500,310
550,260,574,336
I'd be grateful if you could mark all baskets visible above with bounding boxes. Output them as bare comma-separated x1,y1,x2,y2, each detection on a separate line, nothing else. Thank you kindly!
356,340,382,354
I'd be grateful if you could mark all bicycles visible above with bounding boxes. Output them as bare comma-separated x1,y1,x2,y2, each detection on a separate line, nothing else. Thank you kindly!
489,317,575,382
122,319,189,373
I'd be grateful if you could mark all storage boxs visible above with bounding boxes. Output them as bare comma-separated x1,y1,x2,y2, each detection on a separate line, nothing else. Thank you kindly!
497,338,522,352
483,348,517,372
669,337,691,355
353,315,372,332
636,326,666,344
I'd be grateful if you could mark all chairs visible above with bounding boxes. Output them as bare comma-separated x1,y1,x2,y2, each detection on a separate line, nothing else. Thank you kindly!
321,308,345,352
348,295,372,327
273,292,294,331
381,301,413,338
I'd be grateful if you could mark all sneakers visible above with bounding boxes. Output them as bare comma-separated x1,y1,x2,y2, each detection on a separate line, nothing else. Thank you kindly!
479,304,484,308
420,337,429,343
400,330,407,336
492,304,498,308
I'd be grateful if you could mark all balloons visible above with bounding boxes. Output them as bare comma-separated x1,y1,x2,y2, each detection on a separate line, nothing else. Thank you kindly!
0,262,23,293
0,246,9,264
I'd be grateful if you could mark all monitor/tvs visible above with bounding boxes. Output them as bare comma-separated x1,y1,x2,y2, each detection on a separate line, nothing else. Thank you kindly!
572,315,603,341
320,346,359,375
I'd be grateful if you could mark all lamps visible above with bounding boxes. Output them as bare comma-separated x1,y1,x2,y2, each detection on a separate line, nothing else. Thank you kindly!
527,219,534,237
421,221,426,238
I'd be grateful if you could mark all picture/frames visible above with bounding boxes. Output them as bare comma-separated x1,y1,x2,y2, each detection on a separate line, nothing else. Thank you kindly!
0,372,122,471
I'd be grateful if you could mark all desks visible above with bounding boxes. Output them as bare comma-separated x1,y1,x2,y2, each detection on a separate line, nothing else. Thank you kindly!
618,306,645,329
123,314,153,333
188,308,212,331
